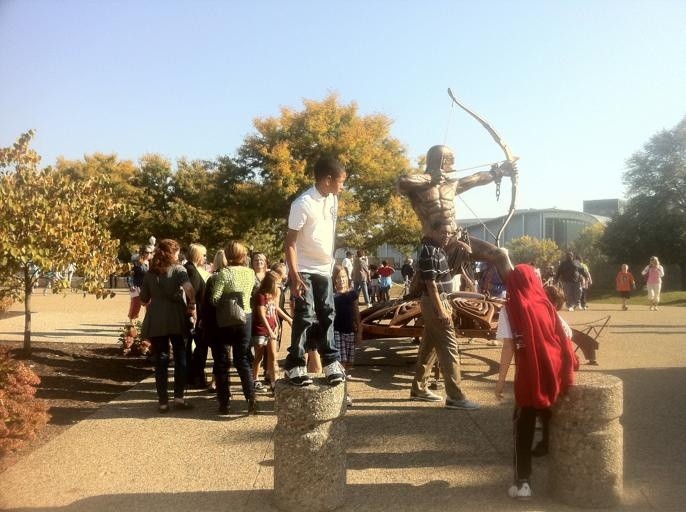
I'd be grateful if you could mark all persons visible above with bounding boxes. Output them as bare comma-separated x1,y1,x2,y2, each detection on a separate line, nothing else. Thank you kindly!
409,217,480,411
281,156,348,386
356,145,523,319
531,285,572,458
28,258,121,297
528,249,593,311
494,262,580,503
641,255,665,312
122,239,414,417
615,263,636,311
328,262,363,409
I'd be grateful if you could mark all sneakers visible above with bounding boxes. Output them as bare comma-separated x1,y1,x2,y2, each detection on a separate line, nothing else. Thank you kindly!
445,395,477,409
284,359,352,406
568,305,588,311
411,390,443,401
533,440,548,456
651,306,659,311
159,380,276,413
508,481,532,497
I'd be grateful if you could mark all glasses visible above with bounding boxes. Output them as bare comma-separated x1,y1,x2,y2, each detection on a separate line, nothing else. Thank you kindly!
144,251,152,254
204,254,206,256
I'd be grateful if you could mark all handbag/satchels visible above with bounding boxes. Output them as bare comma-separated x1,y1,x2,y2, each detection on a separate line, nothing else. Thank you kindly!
216,299,247,327
